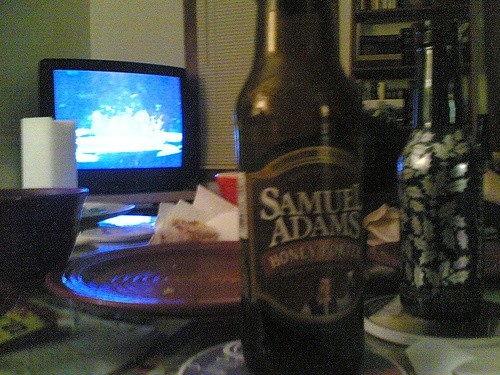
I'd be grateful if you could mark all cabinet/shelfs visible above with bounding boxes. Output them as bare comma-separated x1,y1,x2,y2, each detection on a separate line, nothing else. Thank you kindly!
349,1,474,128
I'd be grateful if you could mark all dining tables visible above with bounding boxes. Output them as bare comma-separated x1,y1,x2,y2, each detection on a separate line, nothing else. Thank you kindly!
0,201,500,373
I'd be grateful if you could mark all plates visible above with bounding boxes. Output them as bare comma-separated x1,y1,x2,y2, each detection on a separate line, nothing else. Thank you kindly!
62,240,241,317
75,226,156,245
178,340,406,375
77,201,137,225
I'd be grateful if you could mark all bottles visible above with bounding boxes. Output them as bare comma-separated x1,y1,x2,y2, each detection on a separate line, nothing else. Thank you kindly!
395,18,490,321
231,0,368,375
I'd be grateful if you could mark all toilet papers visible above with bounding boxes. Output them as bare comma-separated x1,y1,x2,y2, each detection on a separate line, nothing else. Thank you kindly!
21,117,81,191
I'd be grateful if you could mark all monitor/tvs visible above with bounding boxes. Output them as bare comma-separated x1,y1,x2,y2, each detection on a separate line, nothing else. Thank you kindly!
39,57,200,192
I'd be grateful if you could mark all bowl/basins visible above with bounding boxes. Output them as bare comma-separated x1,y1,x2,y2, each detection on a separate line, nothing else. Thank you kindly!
0,185,87,290
